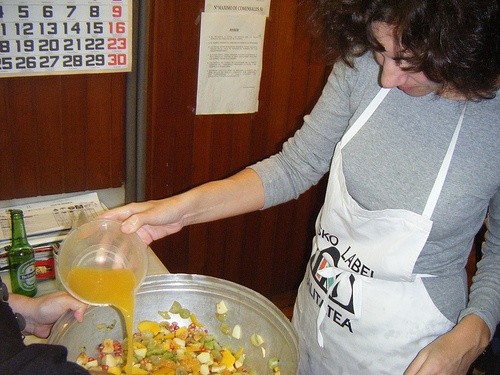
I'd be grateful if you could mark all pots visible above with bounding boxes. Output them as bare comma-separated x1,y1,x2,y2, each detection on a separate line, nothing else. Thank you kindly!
46,273,302,375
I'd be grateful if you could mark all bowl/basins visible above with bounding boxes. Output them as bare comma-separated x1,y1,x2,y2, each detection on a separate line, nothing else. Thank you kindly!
56,219,148,306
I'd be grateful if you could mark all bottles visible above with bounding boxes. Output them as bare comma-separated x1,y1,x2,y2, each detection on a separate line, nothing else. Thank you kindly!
7,209,37,296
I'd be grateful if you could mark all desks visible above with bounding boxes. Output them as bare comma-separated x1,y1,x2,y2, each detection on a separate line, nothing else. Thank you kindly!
0,247,172,346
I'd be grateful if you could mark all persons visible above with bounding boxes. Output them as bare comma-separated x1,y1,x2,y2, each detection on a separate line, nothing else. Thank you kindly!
75,0,500,374
0,275,88,375
